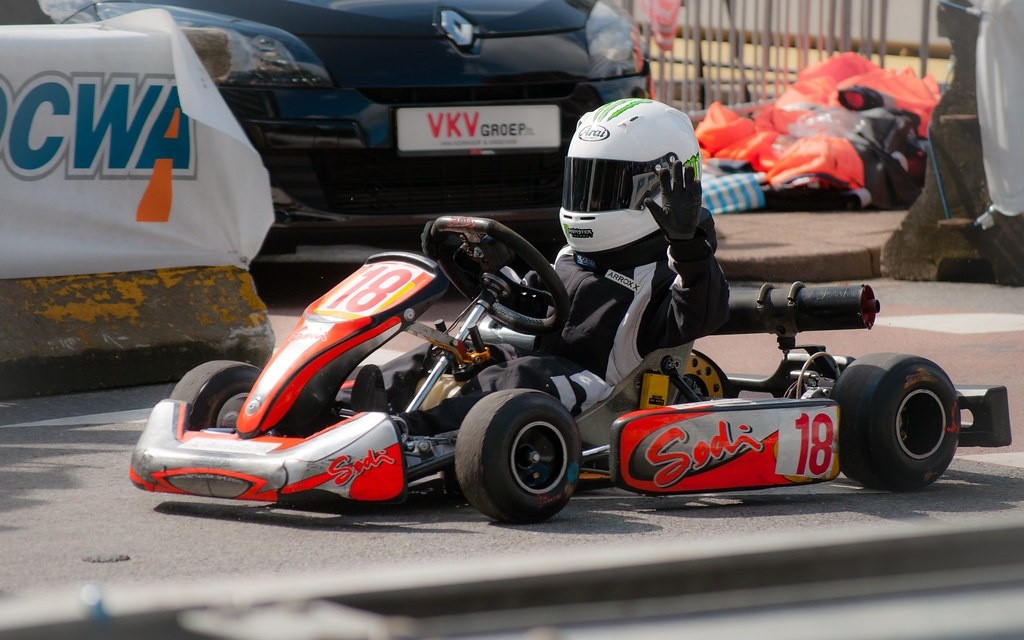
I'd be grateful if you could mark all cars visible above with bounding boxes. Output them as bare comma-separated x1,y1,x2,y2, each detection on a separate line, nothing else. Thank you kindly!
0,0,656,303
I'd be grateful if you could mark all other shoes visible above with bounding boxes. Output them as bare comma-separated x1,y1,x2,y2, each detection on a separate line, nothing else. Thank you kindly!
350,364,390,417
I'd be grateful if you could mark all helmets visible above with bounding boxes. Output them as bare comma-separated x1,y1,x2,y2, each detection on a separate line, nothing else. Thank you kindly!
559,97,701,252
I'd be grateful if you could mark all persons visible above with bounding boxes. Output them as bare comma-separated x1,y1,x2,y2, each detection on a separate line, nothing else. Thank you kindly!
335,96,733,445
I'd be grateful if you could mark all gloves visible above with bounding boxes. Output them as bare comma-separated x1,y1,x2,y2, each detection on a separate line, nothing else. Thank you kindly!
421,220,464,260
644,161,703,240
